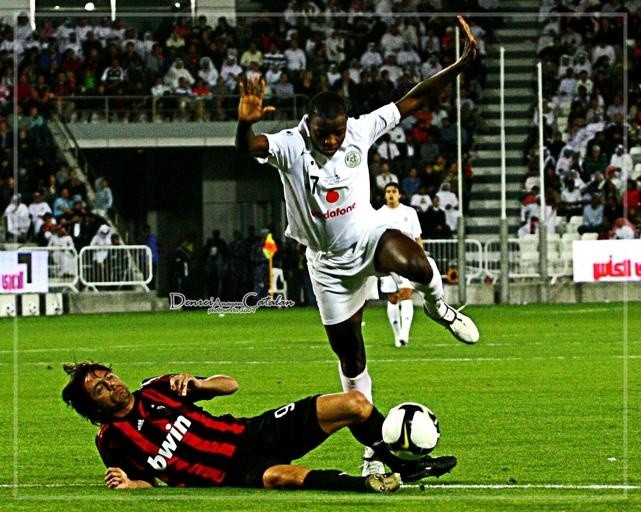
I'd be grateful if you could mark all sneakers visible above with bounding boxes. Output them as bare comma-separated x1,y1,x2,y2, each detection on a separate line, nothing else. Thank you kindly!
395,333,409,347
400,456,458,484
365,472,403,494
423,302,481,344
361,446,386,475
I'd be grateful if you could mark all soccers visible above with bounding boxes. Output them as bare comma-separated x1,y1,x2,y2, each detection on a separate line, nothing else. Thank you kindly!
382,403,440,461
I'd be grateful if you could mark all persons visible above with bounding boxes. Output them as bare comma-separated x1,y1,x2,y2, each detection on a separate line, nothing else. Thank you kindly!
61,359,457,493
236,39,480,478
376,182,423,348
